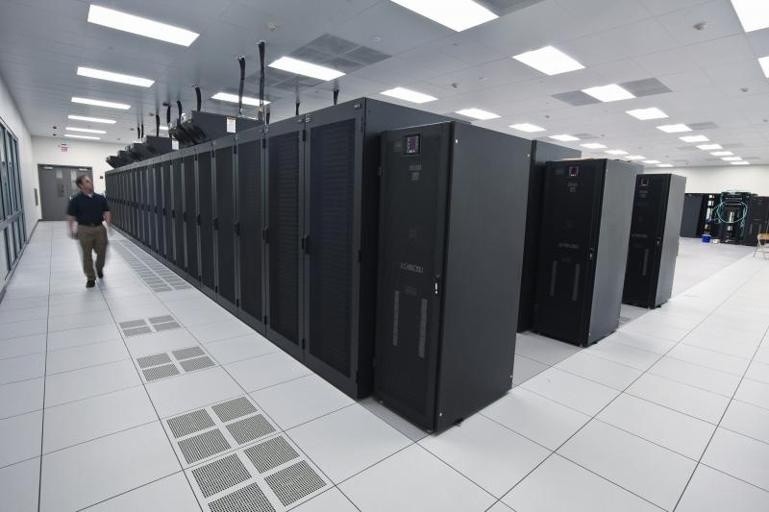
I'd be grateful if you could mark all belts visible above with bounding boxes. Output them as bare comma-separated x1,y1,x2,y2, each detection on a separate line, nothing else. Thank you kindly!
79,222,102,228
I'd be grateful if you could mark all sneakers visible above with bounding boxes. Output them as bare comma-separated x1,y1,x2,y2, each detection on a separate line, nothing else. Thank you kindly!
86,270,104,287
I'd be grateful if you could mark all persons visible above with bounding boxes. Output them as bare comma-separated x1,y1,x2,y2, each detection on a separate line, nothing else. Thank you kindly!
66,173,112,289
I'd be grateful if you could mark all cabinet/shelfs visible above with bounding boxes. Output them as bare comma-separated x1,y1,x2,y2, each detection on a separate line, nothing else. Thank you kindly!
517,138,582,336
106,95,471,402
622,173,687,311
531,157,636,347
373,120,533,437
680,192,768,246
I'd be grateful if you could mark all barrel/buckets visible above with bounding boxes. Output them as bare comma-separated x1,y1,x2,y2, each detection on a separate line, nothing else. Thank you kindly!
702,233,712,243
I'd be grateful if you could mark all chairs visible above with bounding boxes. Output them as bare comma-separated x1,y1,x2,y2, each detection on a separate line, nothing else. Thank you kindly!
753,232,768,260
